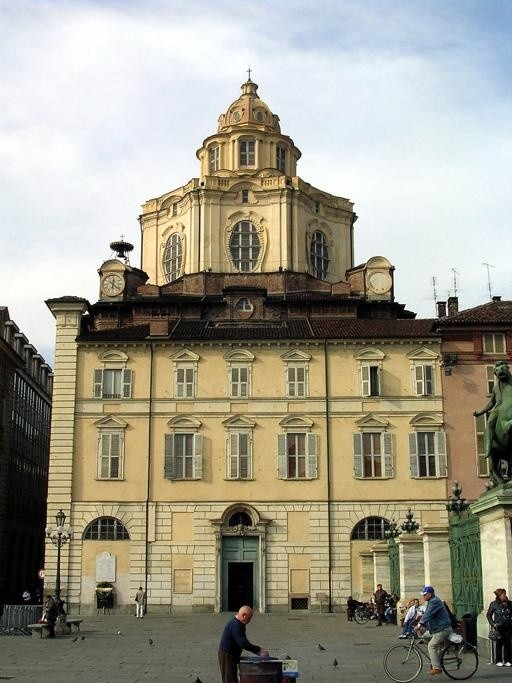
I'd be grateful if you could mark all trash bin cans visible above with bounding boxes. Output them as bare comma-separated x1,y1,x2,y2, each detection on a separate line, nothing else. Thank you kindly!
461,613,477,653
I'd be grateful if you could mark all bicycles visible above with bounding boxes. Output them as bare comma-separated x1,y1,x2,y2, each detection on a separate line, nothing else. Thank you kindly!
355,597,377,625
383,619,478,683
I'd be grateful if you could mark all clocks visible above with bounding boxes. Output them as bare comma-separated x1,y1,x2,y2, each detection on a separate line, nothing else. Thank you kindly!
100,273,125,297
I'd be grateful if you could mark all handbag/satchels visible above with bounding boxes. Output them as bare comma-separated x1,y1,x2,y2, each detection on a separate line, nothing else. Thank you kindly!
488,631,501,641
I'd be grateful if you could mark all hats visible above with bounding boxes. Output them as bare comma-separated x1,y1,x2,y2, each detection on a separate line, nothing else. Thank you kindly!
421,587,435,595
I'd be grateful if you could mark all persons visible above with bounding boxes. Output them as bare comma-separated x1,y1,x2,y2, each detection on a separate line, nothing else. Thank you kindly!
22,588,66,623
135,586,145,619
346,583,457,644
487,588,512,667
413,586,453,676
216,605,269,683
45,595,58,638
473,382,502,460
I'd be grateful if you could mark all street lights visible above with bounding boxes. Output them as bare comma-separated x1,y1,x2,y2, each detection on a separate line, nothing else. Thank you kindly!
45,510,74,597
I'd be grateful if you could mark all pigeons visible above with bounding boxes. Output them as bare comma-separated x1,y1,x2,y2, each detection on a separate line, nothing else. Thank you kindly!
317,644,326,652
117,630,122,635
332,658,338,668
148,638,153,644
80,636,85,640
193,676,203,683
286,656,291,659
71,637,78,643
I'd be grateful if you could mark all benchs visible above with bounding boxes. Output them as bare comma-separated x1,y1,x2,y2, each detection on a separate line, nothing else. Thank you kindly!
27,618,83,639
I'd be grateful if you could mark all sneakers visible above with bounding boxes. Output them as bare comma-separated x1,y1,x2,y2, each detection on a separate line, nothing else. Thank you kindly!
398,634,406,638
495,662,511,667
376,623,382,626
426,668,441,675
